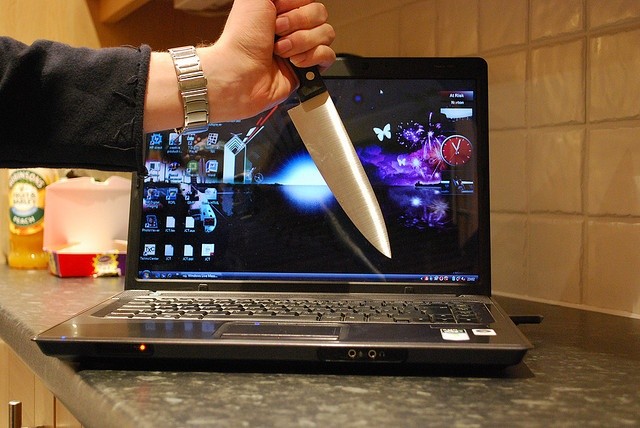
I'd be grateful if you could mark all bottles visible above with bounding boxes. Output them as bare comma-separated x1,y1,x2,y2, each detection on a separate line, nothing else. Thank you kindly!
8,169,60,269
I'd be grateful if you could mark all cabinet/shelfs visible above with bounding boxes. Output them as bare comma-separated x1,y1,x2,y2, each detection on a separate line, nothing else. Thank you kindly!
0,341,81,427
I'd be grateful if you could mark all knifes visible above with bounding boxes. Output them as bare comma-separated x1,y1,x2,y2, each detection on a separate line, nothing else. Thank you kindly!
287,64,392,260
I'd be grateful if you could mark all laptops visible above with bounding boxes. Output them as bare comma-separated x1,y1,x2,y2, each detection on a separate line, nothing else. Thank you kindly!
29,56,536,367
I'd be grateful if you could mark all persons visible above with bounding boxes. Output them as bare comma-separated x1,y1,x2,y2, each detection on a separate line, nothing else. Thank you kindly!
0,0,337,173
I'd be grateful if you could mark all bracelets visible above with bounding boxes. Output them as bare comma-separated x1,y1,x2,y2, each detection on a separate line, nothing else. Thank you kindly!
166,46,209,135
